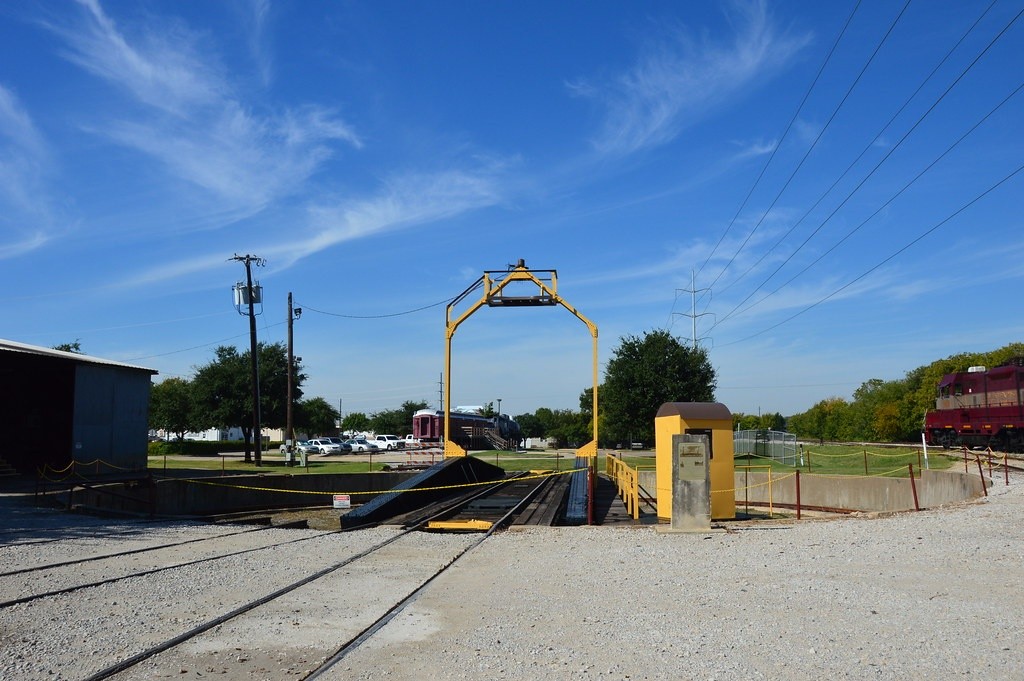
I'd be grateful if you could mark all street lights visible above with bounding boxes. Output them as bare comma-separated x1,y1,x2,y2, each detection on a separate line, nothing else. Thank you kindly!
497,399,502,436
285,291,302,468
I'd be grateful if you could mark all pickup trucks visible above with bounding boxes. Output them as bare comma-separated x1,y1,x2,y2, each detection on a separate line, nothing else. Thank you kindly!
366,434,407,451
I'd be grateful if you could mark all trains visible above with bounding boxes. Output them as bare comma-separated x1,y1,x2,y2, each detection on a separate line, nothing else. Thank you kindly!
925,355,1024,452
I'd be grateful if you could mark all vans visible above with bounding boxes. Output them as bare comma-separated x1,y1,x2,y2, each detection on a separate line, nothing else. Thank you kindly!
406,434,423,444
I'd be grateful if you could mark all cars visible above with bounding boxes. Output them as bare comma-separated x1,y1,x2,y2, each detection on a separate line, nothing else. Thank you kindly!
279,440,319,458
306,439,342,456
345,438,379,455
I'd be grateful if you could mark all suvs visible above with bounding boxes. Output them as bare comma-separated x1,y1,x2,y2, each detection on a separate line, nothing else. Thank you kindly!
318,436,353,455
